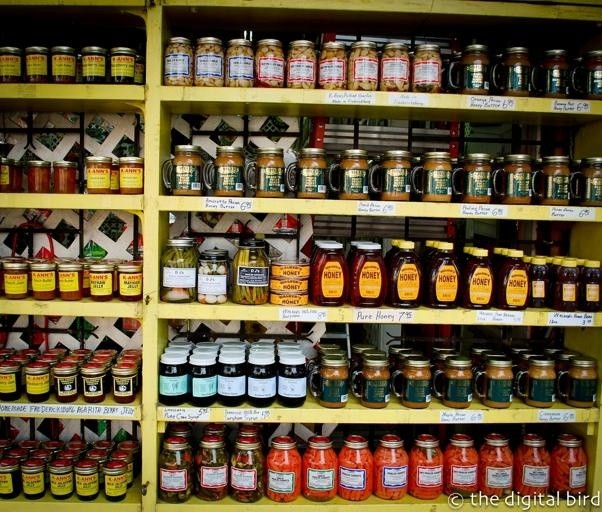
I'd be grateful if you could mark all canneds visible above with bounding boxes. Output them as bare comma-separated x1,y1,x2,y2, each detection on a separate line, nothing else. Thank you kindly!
0,349,142,403
0,45,146,85
163,37,602,100
157,424,588,504
307,343,597,407
0,440,141,502
159,237,311,306
0,155,144,194
163,145,602,207
0,255,143,302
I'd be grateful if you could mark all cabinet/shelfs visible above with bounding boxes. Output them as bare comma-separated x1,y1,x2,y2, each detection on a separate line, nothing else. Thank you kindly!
0,0,601,512
